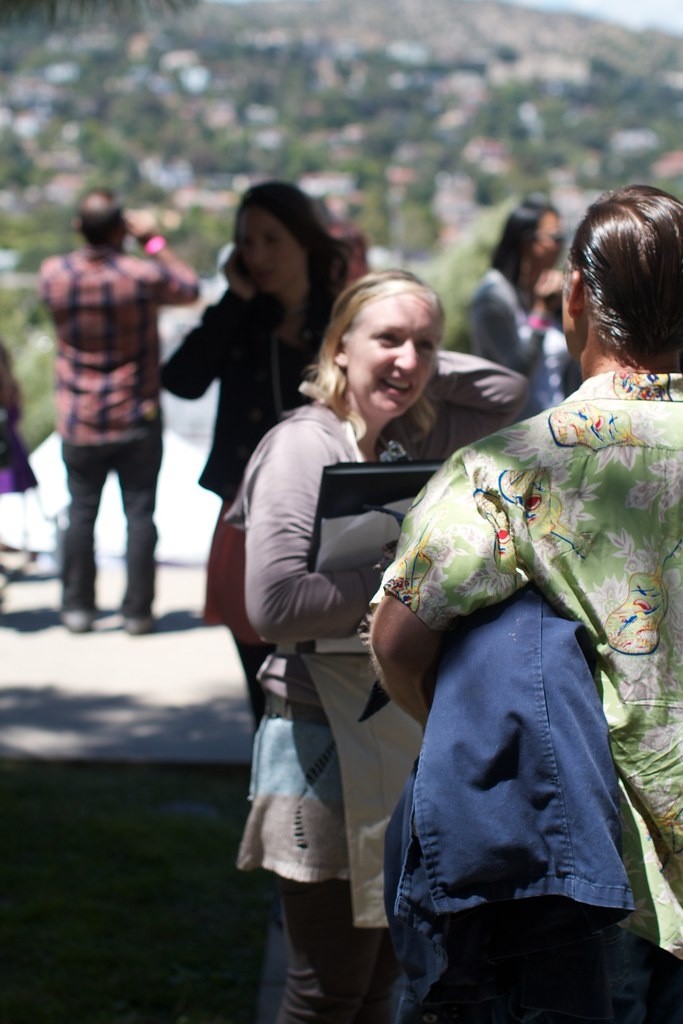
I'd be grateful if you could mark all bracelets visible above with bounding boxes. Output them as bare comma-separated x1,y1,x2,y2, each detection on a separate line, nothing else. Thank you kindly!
145,235,165,253
528,315,552,328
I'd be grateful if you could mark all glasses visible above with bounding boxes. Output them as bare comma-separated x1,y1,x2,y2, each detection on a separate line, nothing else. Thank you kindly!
540,232,565,246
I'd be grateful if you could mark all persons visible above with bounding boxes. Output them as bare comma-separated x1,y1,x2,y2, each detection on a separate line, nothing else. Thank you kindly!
0,343,38,497
159,182,350,734
38,188,200,628
221,269,530,1024
368,185,683,1024
464,196,572,421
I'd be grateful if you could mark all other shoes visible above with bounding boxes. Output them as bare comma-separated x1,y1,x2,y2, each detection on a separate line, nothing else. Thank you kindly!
125,614,152,634
59,607,95,633
